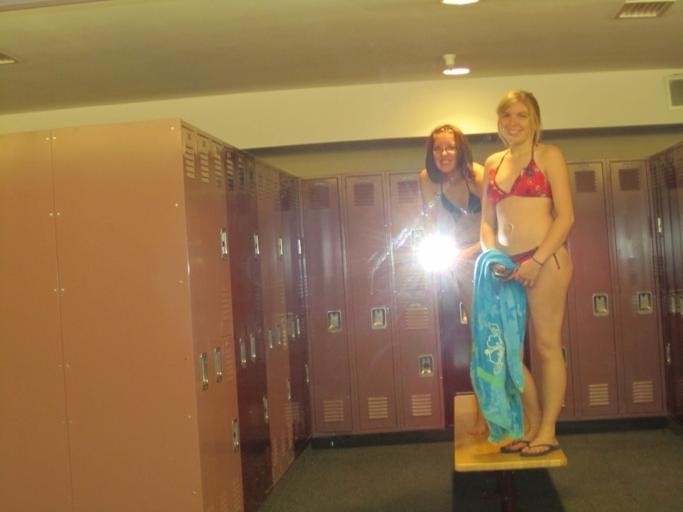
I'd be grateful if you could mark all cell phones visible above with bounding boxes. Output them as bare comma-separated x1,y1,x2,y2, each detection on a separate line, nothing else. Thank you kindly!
495,268,516,277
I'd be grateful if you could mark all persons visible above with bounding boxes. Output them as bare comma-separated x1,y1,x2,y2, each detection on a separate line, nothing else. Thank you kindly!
417,123,502,438
478,86,577,459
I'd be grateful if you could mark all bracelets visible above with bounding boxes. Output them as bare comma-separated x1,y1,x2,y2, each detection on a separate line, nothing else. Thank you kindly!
530,255,543,268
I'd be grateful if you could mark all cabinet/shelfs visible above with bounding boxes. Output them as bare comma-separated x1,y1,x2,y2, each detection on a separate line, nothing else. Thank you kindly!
0,117,683,512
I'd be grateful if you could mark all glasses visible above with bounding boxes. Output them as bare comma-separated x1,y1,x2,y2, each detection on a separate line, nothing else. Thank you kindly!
432,145,456,152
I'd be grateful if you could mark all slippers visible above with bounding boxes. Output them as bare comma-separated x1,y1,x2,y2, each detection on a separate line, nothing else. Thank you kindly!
501,439,561,457
